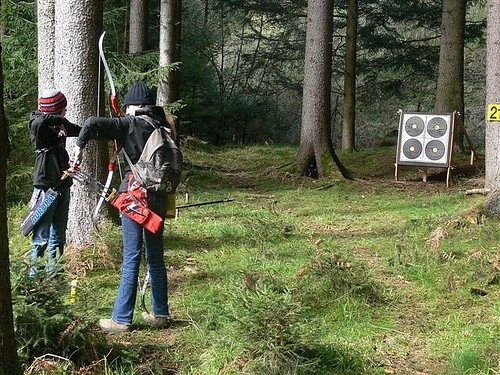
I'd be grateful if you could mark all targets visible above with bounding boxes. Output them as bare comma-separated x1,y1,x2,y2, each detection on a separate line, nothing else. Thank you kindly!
405,116,425,137
403,138,422,159
425,139,445,160
427,117,447,138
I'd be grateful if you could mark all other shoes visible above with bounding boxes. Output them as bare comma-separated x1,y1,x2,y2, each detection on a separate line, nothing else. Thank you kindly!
141,312,168,328
98,318,132,332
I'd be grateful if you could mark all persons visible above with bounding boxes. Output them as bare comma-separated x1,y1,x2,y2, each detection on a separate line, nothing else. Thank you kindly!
28,88,110,280
68,82,177,332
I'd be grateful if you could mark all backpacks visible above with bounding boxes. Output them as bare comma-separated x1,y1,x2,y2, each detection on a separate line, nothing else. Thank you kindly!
132,126,183,196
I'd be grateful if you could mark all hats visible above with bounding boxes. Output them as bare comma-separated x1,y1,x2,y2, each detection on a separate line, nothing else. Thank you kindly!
124,82,154,105
39,89,67,114
